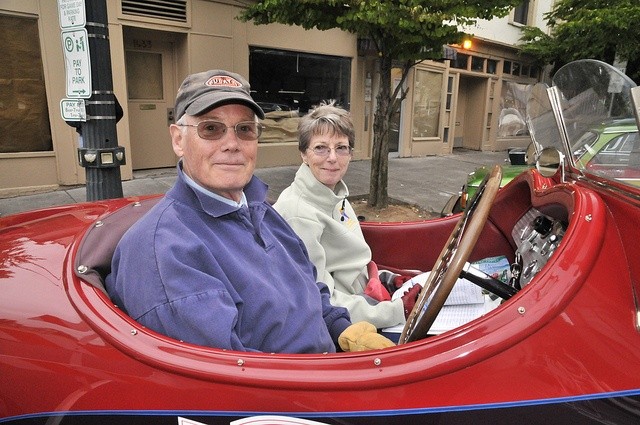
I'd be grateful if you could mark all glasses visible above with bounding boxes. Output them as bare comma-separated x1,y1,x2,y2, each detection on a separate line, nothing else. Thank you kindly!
307,144,354,156
178,120,265,141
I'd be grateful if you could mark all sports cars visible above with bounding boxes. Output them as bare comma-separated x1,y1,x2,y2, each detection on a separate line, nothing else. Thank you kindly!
3,60,638,425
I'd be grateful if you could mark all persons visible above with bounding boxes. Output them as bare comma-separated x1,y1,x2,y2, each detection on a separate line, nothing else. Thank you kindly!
272,99,434,344
104,69,396,354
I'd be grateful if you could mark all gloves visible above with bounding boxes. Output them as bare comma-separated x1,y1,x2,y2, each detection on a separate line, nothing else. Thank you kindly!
337,321,397,352
393,275,414,289
401,283,423,321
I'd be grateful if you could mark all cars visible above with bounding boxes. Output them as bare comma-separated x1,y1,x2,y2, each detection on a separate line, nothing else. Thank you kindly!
449,117,638,217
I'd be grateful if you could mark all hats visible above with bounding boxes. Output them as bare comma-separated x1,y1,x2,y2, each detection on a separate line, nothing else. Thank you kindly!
174,69,265,122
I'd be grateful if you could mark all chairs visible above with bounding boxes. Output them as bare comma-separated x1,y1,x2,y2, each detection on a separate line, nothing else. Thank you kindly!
73,194,167,298
